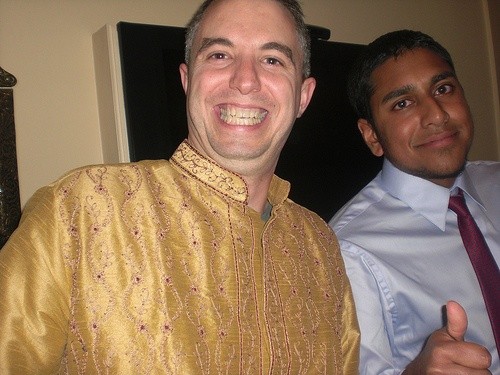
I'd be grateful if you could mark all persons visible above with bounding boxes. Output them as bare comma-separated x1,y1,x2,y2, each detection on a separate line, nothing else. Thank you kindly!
0,0,361,375
328,29,500,375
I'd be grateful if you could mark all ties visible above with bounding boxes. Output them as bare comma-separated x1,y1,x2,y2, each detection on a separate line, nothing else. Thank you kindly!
448,187,500,360
